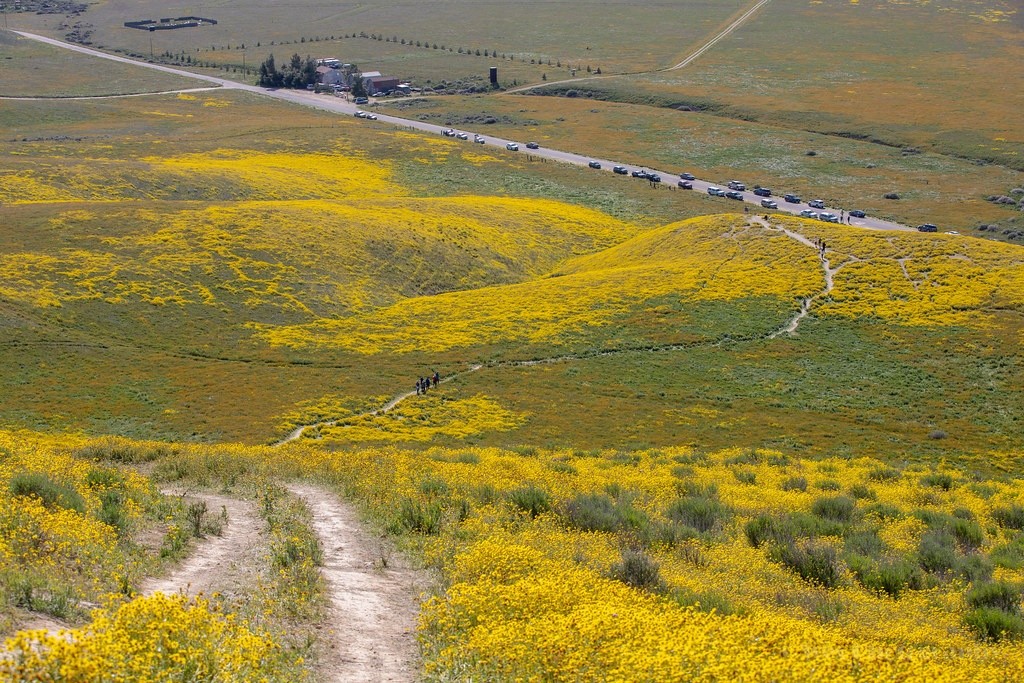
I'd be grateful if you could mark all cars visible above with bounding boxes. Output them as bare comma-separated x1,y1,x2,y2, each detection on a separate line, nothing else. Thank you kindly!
726,191,743,200
680,173,695,180
945,231,961,235
848,210,866,218
588,160,601,170
646,172,660,182
784,194,801,203
631,170,646,178
303,79,397,121
800,209,818,219
613,166,628,174
761,199,777,209
441,128,540,152
706,186,725,197
678,180,692,189
917,223,937,233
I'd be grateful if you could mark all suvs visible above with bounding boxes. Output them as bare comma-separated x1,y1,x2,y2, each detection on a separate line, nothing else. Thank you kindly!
752,187,771,197
727,181,745,191
808,199,824,208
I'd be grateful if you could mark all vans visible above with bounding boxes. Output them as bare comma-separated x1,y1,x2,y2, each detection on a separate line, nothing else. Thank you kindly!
819,212,838,223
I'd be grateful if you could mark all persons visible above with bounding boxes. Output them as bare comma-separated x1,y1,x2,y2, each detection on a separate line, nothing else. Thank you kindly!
839,208,851,224
814,238,826,258
416,371,439,395
